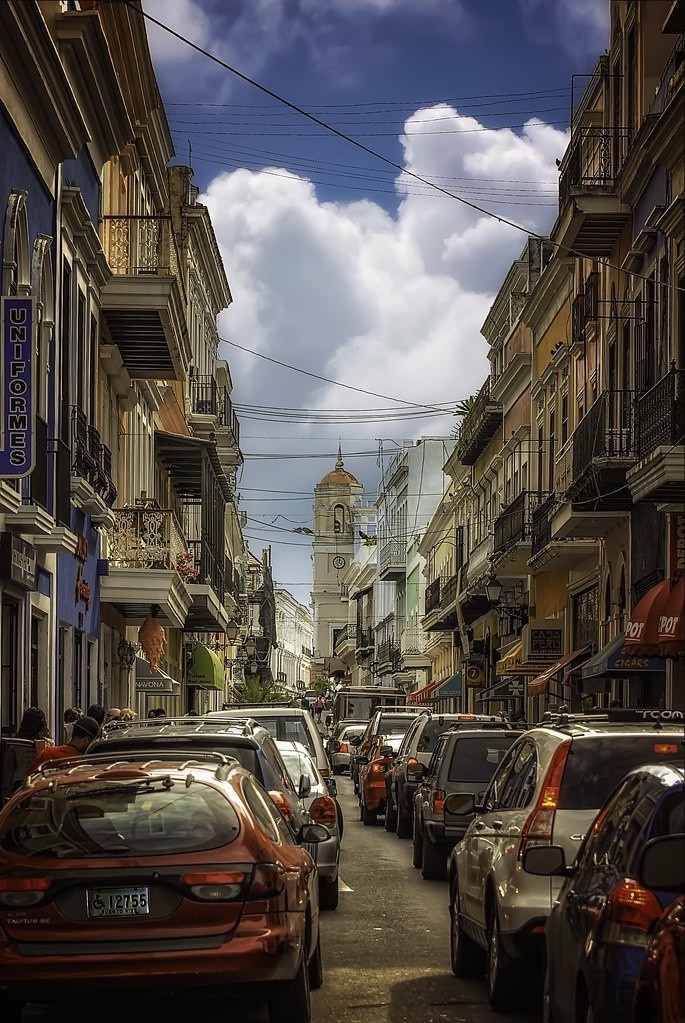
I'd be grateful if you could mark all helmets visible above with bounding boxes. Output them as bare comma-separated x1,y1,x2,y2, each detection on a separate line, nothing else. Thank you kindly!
72,716,99,737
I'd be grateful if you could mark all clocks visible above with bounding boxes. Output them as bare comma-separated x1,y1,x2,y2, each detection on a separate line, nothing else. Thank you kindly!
332,556,345,569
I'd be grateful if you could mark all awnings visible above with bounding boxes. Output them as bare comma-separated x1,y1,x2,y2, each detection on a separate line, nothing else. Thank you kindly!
411,577,685,704
186,647,224,690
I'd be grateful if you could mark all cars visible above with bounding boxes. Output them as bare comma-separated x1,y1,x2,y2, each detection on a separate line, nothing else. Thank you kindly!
274,741,343,908
623,834,685,1023
0,745,332,1023
202,679,450,835
523,758,685,1023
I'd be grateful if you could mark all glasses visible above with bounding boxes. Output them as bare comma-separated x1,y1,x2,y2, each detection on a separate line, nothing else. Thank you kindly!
107,713,115,718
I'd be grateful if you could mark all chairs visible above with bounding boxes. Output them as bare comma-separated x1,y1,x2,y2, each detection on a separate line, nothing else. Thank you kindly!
54,805,123,855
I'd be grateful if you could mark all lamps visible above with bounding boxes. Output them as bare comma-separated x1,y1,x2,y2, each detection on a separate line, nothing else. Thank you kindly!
113,634,128,669
483,570,528,623
203,618,258,676
123,642,136,672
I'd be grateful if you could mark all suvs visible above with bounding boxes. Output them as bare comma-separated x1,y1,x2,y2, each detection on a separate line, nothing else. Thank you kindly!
80,714,322,871
379,710,515,839
403,718,533,881
444,704,685,1015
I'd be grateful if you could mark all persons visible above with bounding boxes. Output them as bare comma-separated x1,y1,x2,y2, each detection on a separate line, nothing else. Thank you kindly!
13,704,214,782
